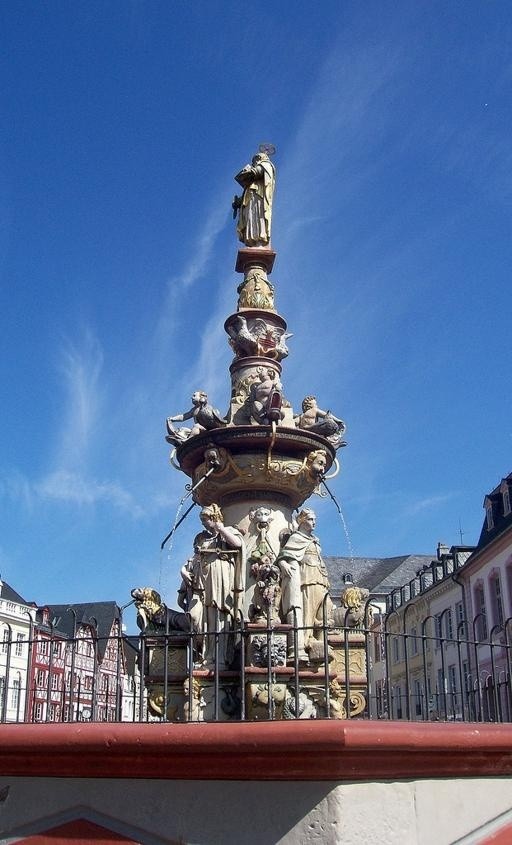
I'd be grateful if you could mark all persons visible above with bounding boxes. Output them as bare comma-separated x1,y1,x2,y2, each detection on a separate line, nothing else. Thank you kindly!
306,449,327,480
203,447,227,474
182,677,206,721
180,531,211,649
196,504,246,665
231,153,275,248
276,506,335,652
162,390,345,444
319,679,346,720
